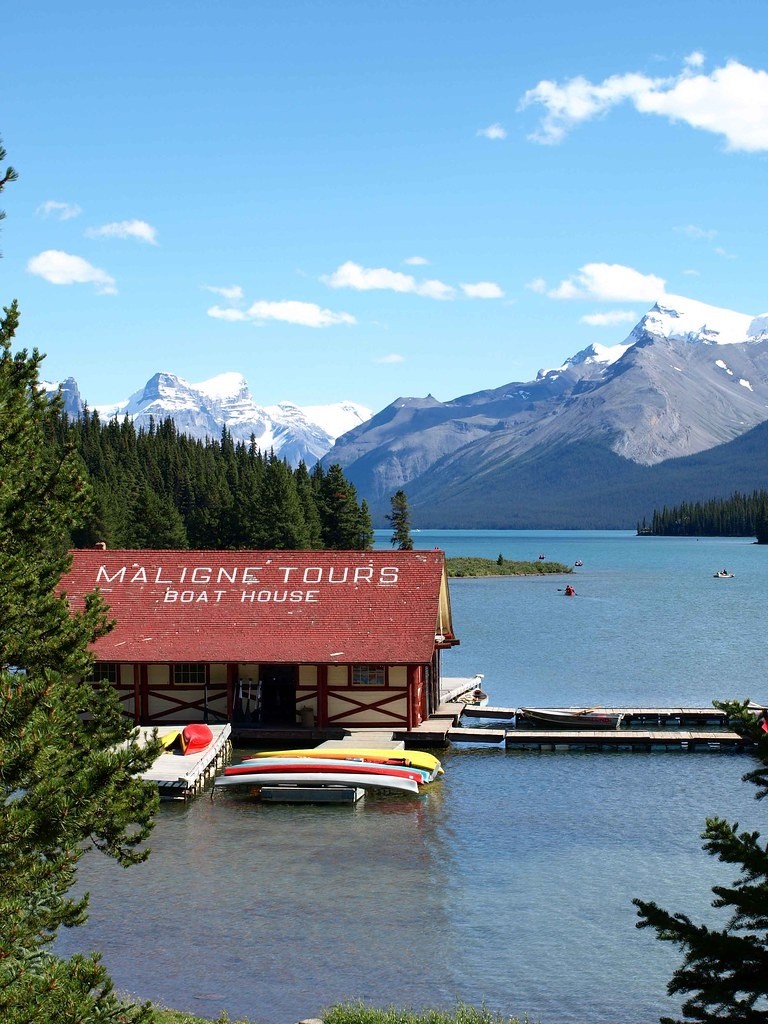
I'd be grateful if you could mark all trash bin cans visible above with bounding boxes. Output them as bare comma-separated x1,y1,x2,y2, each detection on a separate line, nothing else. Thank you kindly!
300,708,314,728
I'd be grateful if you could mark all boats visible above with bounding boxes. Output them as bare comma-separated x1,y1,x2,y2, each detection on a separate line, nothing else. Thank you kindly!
563,586,576,597
717,571,736,579
574,560,584,567
210,748,445,795
539,555,546,560
181,724,213,757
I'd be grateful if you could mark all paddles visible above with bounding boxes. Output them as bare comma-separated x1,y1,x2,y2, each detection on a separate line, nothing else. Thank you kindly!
573,591,577,595
235,678,263,723
557,589,566,591
